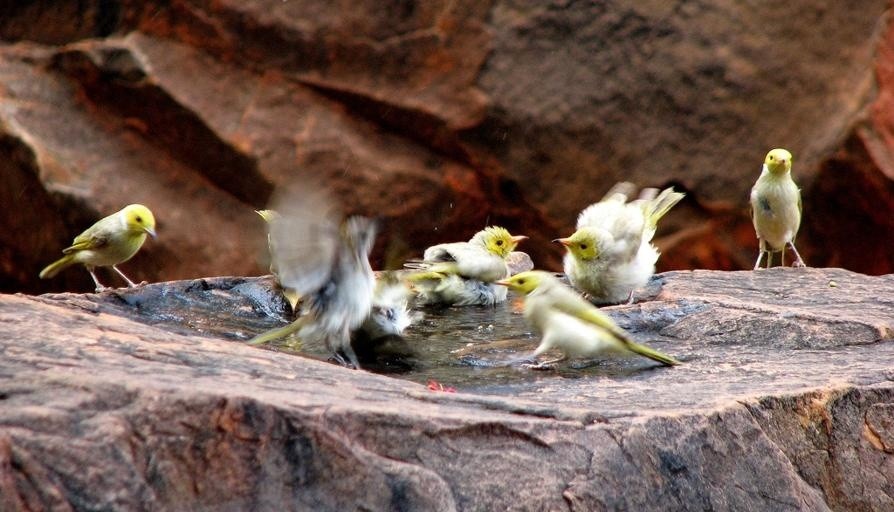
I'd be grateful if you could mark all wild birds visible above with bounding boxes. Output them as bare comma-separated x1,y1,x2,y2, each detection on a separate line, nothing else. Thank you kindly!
244,166,416,371
551,181,687,306
494,269,684,371
397,225,530,307
748,148,806,271
39,204,158,294
